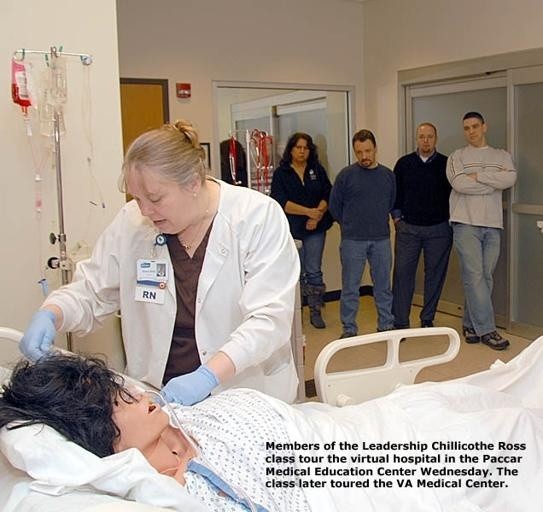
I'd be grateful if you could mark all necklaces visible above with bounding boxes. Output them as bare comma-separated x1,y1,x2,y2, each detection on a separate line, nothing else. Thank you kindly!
177,205,210,250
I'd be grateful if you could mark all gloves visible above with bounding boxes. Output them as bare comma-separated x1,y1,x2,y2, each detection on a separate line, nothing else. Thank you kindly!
160,366,218,406
20,310,56,361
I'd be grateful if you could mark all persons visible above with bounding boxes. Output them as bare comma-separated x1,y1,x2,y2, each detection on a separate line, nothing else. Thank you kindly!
330,128,394,339
0,354,313,512
18,121,299,409
446,111,516,351
392,122,453,342
270,132,332,329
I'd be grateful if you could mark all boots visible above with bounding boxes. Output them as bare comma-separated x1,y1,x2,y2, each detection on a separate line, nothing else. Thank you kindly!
305,283,326,330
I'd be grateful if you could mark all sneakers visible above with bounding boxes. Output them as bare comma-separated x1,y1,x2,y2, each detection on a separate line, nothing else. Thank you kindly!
481,330,509,350
463,321,480,345
